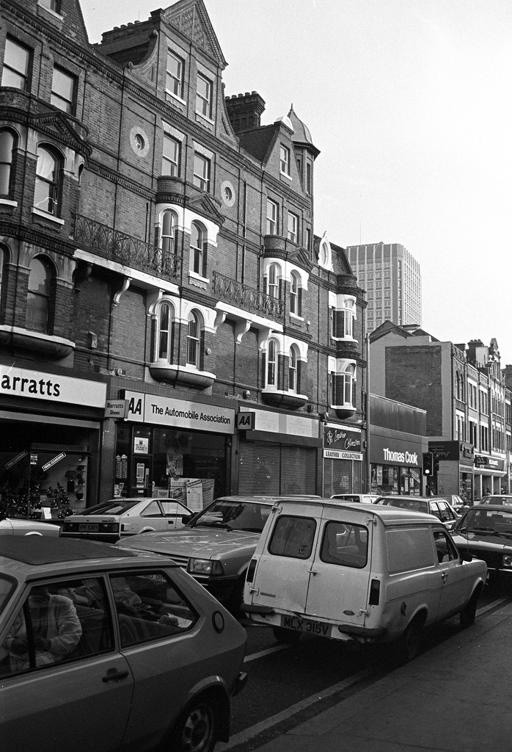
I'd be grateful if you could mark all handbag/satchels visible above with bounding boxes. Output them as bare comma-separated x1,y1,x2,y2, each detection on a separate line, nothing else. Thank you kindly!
76,489,83,500
76,466,84,485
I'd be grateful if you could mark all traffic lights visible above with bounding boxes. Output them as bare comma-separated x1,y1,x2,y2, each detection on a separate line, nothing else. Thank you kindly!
421,452,434,476
432,456,440,472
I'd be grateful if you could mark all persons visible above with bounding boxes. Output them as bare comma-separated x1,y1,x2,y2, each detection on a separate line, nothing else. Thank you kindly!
1,586,82,674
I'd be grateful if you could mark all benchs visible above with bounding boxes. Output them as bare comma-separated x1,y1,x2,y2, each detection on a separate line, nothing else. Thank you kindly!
75,604,181,656
